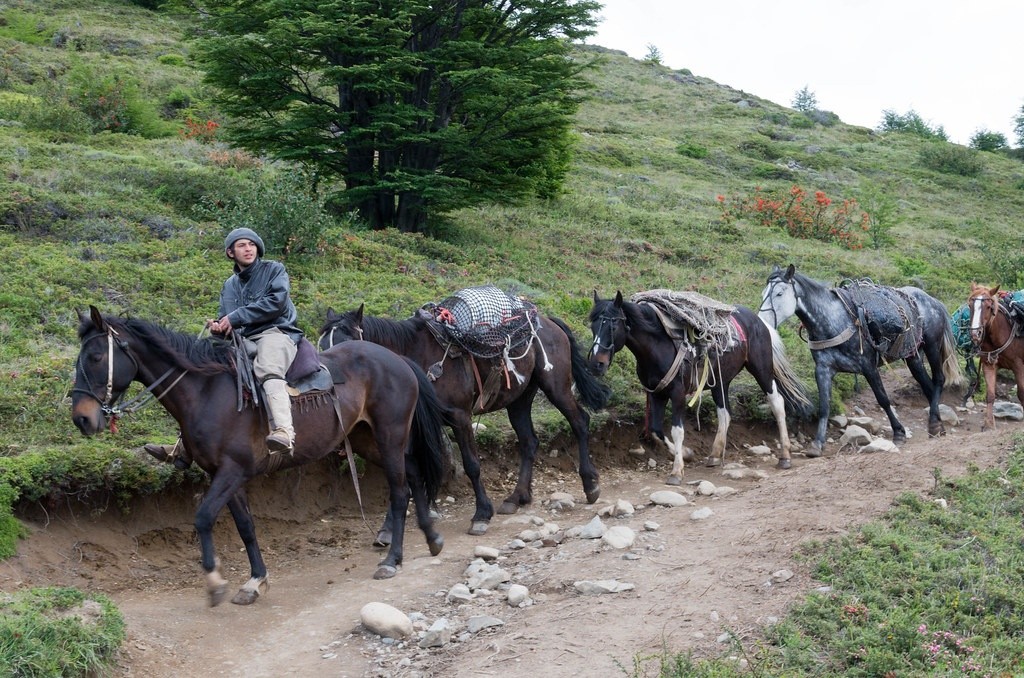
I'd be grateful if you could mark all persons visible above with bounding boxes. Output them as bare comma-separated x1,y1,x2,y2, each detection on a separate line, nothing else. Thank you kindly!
145,227,304,471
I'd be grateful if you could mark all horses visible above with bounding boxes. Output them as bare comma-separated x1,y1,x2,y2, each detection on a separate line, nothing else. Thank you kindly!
66,263,1024,608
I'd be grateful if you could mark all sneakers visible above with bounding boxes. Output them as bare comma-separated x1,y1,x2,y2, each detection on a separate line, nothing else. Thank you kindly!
144,439,194,471
265,428,296,459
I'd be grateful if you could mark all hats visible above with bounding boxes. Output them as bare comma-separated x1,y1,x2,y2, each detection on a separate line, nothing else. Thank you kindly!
225,227,265,257
